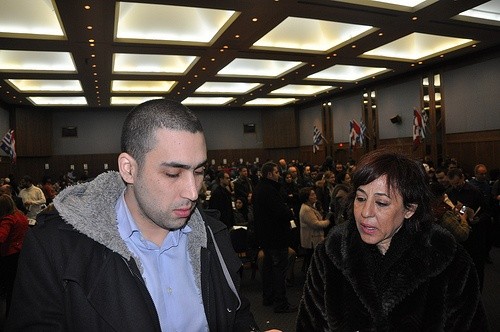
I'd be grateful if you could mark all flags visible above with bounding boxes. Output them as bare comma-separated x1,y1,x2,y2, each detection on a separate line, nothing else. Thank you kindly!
0,129,16,167
313,126,323,153
413,107,430,151
349,120,369,154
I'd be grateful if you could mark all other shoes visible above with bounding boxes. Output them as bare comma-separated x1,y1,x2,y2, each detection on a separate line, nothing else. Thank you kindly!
263,298,273,305
273,303,298,313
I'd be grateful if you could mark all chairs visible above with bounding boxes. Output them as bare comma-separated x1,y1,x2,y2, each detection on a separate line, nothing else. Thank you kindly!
229,225,256,280
287,229,313,282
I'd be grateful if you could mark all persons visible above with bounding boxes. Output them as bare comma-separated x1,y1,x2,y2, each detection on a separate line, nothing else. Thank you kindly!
420,159,500,295
295,149,489,332
199,159,355,312
9,99,282,332
0,177,83,255
